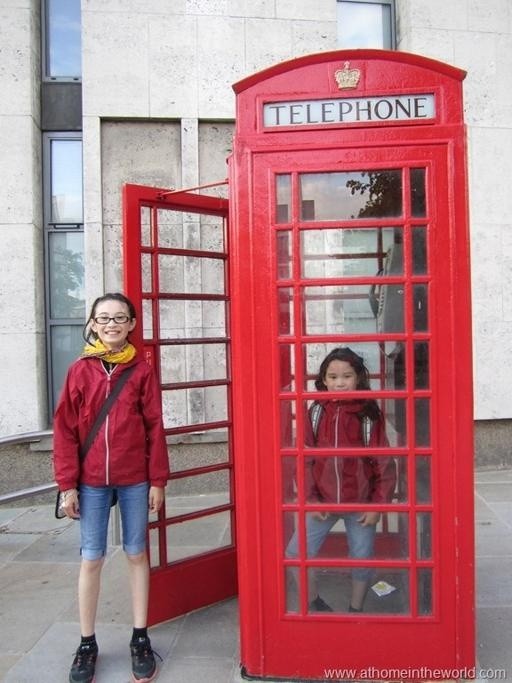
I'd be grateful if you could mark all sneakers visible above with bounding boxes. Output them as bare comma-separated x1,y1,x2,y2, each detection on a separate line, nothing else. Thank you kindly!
349,606,363,613
309,594,334,612
129,640,158,683
69,644,98,683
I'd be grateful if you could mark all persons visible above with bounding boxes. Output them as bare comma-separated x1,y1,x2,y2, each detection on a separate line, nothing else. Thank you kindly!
53,293,169,683
284,347,395,614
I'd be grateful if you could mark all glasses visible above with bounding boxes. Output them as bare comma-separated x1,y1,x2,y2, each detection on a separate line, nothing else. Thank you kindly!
92,316,132,324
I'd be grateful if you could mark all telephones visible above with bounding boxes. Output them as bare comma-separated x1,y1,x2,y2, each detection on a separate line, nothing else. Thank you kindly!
369,244,403,332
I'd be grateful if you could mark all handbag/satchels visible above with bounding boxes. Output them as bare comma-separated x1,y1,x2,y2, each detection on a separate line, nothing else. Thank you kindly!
55,489,117,521
